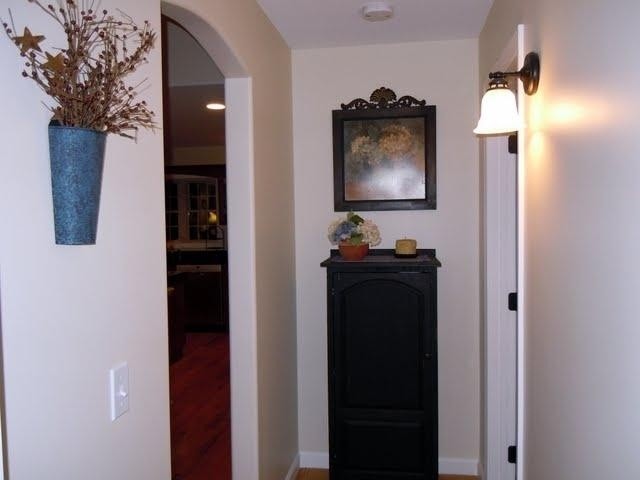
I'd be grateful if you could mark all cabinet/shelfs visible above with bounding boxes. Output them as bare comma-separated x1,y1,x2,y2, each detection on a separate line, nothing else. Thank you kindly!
319,246,442,480
176,252,228,333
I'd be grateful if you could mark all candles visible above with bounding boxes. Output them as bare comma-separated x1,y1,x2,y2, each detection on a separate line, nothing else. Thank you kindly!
396,239,417,255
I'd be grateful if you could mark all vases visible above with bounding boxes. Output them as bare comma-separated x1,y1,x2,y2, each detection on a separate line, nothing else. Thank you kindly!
338,243,370,262
48,125,107,247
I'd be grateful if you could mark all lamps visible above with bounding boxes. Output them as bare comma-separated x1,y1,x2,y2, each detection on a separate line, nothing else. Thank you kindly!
359,2,395,23
471,51,541,138
205,96,226,110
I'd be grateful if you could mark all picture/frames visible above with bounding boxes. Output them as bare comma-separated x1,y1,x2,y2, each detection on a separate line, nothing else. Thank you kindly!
332,86,438,212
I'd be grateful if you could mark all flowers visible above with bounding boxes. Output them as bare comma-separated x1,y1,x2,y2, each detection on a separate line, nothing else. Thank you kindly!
327,208,382,249
